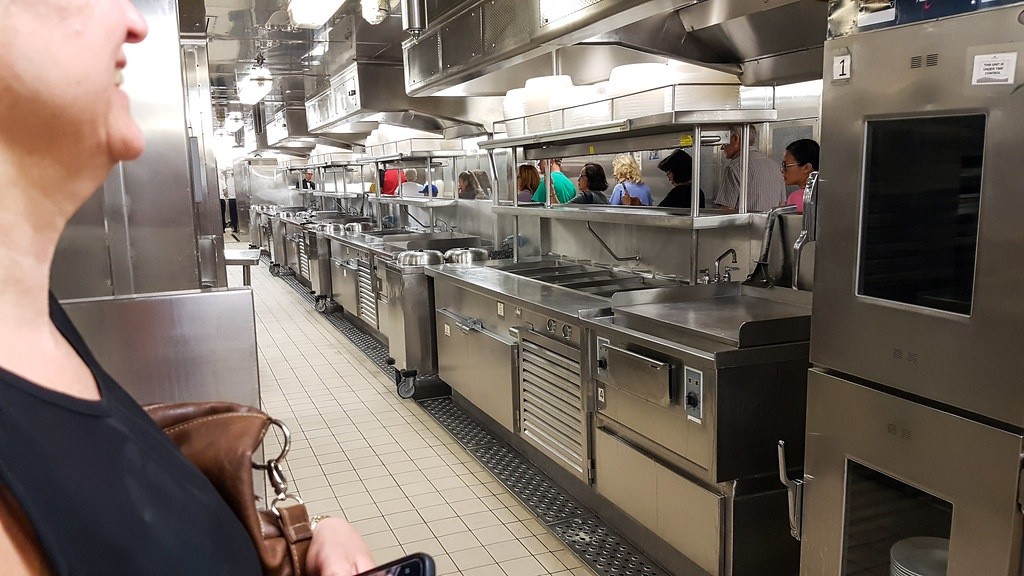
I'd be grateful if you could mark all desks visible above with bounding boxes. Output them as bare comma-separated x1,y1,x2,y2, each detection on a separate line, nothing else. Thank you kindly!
224,247,263,286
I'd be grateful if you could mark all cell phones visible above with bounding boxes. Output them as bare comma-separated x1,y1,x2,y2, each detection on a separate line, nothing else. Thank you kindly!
354,552,436,576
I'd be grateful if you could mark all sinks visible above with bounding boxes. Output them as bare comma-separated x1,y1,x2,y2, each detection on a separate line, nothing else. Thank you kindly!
358,229,425,238
386,232,482,250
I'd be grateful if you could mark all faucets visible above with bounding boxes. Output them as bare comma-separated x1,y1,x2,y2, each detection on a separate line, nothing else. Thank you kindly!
698,248,741,284
433,217,448,232
310,199,320,211
347,205,359,217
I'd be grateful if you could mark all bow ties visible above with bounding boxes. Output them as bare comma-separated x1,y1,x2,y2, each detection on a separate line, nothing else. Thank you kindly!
306,180,311,183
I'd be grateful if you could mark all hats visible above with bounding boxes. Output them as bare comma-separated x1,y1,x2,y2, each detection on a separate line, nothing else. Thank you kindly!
418,184,438,194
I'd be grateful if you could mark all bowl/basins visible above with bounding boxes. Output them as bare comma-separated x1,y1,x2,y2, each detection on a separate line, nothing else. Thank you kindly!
307,59,741,166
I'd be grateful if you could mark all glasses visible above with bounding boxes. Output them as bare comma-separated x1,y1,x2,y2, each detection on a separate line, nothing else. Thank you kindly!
779,162,801,173
665,171,672,180
578,173,586,178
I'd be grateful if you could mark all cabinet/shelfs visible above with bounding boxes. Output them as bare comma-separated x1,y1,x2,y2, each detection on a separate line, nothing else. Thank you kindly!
286,150,466,234
477,108,779,287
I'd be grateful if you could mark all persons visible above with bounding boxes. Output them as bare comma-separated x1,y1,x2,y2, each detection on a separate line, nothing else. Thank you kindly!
296,169,315,190
382,169,407,195
566,162,610,204
225,173,239,233
780,139,820,212
517,164,540,202
714,125,787,212
393,168,424,196
656,148,706,208
530,158,577,203
454,171,488,199
0,0,379,576
609,154,654,206
473,169,492,195
419,184,438,197
370,168,384,194
218,178,228,234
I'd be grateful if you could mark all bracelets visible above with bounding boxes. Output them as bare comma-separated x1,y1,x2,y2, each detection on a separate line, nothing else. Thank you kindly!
309,515,330,530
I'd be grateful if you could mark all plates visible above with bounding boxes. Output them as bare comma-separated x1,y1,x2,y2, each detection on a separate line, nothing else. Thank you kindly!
889,535,949,576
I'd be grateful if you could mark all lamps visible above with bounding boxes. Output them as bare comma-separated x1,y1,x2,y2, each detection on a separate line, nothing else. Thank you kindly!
224,112,244,133
288,0,344,28
237,67,275,106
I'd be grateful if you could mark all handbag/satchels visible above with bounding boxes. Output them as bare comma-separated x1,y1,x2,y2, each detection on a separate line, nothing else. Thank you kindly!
140,398,313,576
621,182,642,206
